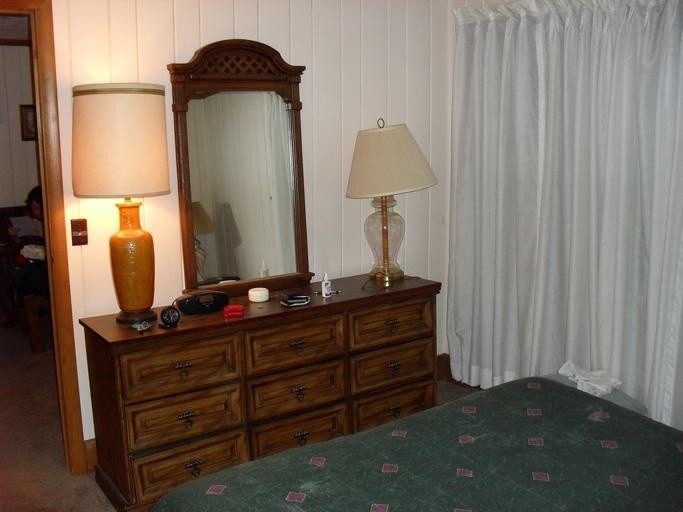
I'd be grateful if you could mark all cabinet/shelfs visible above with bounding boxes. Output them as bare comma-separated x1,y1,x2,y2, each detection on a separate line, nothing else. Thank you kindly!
79,271,442,512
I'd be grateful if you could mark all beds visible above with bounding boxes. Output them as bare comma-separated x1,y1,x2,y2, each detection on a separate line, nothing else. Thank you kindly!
151,378,683,511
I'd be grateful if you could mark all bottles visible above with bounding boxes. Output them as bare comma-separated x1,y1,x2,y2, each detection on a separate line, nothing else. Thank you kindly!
321,272,332,297
261,260,268,277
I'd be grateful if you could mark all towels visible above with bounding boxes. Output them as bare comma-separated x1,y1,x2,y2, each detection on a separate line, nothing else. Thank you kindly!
557,359,622,399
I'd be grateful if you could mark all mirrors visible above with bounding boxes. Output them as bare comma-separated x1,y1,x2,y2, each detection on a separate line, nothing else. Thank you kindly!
166,39,315,298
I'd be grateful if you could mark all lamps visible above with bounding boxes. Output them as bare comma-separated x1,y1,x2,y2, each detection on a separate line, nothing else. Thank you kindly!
71,82,172,325
192,201,217,286
346,118,438,282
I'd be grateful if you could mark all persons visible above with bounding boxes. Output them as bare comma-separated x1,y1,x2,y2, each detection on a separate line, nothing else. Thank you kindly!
0,184,48,332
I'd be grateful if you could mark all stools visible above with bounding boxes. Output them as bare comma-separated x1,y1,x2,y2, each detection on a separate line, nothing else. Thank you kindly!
543,373,648,416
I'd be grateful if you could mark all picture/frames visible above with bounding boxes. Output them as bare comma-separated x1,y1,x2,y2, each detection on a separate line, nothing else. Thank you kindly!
20,104,38,141
